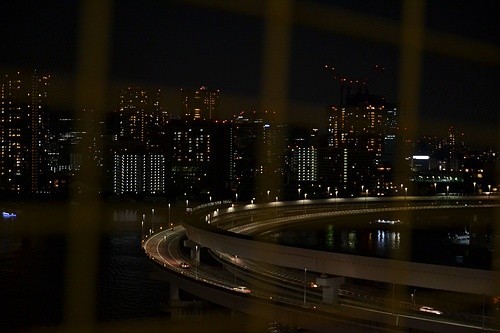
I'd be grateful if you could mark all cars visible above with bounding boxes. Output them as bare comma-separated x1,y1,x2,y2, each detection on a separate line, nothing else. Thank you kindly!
231,286,251,294
180,262,190,269
